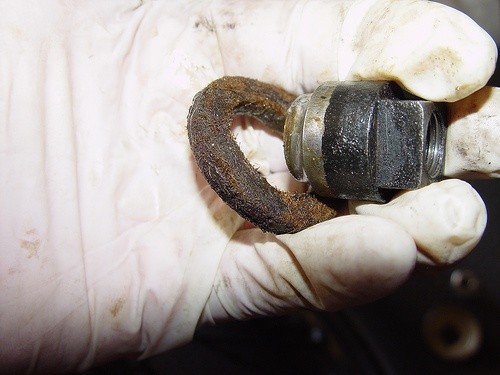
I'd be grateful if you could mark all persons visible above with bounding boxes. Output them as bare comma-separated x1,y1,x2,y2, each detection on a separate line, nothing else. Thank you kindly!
1,0,499,370
